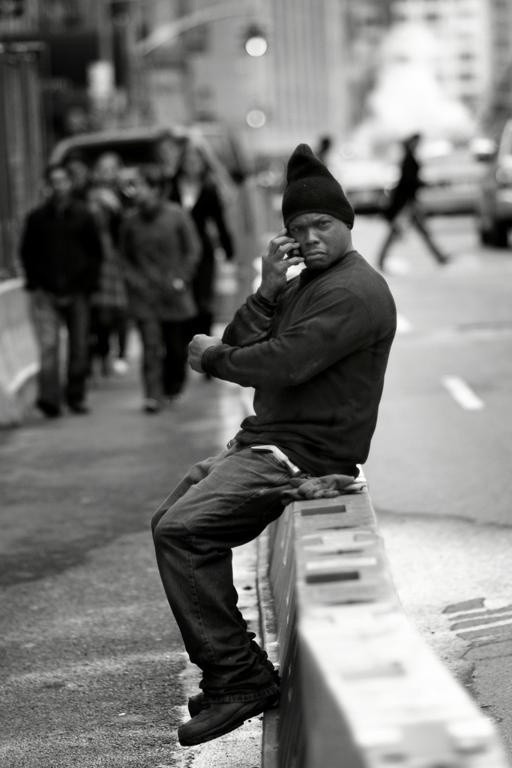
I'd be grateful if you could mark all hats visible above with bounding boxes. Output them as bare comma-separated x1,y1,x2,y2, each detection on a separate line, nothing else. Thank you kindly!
282,144,354,226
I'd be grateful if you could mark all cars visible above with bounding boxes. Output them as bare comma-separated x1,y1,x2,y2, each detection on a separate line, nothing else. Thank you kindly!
470,114,512,248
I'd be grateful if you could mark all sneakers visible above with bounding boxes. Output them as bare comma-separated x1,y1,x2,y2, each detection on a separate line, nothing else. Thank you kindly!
146,398,164,411
178,678,284,748
34,397,61,416
68,401,88,414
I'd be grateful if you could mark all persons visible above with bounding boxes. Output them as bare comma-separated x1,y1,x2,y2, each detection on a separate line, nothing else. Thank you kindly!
147,142,398,748
315,138,333,165
379,131,456,270
15,136,239,422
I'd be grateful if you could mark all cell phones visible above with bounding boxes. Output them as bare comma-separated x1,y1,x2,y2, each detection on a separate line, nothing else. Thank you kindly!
284,233,302,258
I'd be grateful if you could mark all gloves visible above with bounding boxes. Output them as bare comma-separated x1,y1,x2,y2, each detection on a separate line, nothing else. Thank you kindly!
283,474,365,504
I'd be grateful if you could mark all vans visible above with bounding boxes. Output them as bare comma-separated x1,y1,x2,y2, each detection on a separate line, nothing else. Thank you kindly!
45,117,267,326
